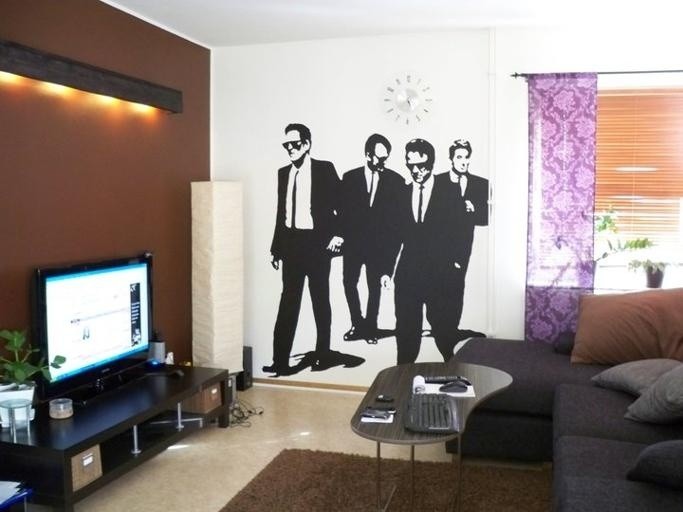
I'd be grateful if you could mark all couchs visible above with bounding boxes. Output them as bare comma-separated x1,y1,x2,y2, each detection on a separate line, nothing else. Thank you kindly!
440,328,683,510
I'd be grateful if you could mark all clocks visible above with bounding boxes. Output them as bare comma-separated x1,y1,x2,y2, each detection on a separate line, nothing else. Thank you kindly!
382,75,434,126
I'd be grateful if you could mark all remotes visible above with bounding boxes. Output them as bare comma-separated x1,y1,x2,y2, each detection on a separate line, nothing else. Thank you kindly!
425,375,471,386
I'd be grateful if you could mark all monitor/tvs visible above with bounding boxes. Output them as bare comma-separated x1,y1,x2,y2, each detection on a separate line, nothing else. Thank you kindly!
36,252,152,407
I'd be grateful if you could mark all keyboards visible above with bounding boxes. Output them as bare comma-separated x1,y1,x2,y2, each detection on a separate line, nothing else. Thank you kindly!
404,393,459,434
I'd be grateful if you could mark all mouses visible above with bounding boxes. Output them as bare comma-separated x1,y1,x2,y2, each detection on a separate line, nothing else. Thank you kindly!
439,381,467,392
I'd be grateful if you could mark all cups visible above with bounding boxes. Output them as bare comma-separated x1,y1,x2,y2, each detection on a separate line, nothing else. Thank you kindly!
49,399,72,419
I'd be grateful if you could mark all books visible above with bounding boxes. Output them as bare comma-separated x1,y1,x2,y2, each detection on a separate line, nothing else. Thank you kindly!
412,375,475,398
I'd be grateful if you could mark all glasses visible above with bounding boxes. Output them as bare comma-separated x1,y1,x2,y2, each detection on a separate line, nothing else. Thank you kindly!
375,154,387,162
406,162,426,171
282,140,301,150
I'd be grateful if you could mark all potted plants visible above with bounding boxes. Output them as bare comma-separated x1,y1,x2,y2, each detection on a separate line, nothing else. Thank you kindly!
0,328,64,430
554,203,668,295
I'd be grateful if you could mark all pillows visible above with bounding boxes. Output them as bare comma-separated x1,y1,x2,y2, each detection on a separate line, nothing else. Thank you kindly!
570,287,681,487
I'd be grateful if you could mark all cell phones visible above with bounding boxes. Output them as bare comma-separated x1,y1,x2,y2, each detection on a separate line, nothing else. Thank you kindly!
360,410,390,419
361,415,394,423
366,407,396,414
376,394,393,402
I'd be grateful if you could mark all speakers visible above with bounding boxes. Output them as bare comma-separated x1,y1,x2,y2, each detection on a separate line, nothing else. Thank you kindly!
229,375,236,405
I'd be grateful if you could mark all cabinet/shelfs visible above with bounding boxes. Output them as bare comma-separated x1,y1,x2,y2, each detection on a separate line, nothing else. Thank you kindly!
0,364,231,510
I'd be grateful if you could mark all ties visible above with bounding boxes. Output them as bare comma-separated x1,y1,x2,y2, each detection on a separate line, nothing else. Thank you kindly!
292,171,298,229
418,185,424,223
368,171,375,204
458,174,460,185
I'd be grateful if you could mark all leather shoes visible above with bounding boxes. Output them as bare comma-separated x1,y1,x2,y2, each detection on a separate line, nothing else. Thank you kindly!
263,362,289,372
365,333,378,344
311,354,328,371
343,325,362,340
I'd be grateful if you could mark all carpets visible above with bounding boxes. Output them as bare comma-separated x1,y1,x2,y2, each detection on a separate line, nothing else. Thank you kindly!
222,446,557,511
